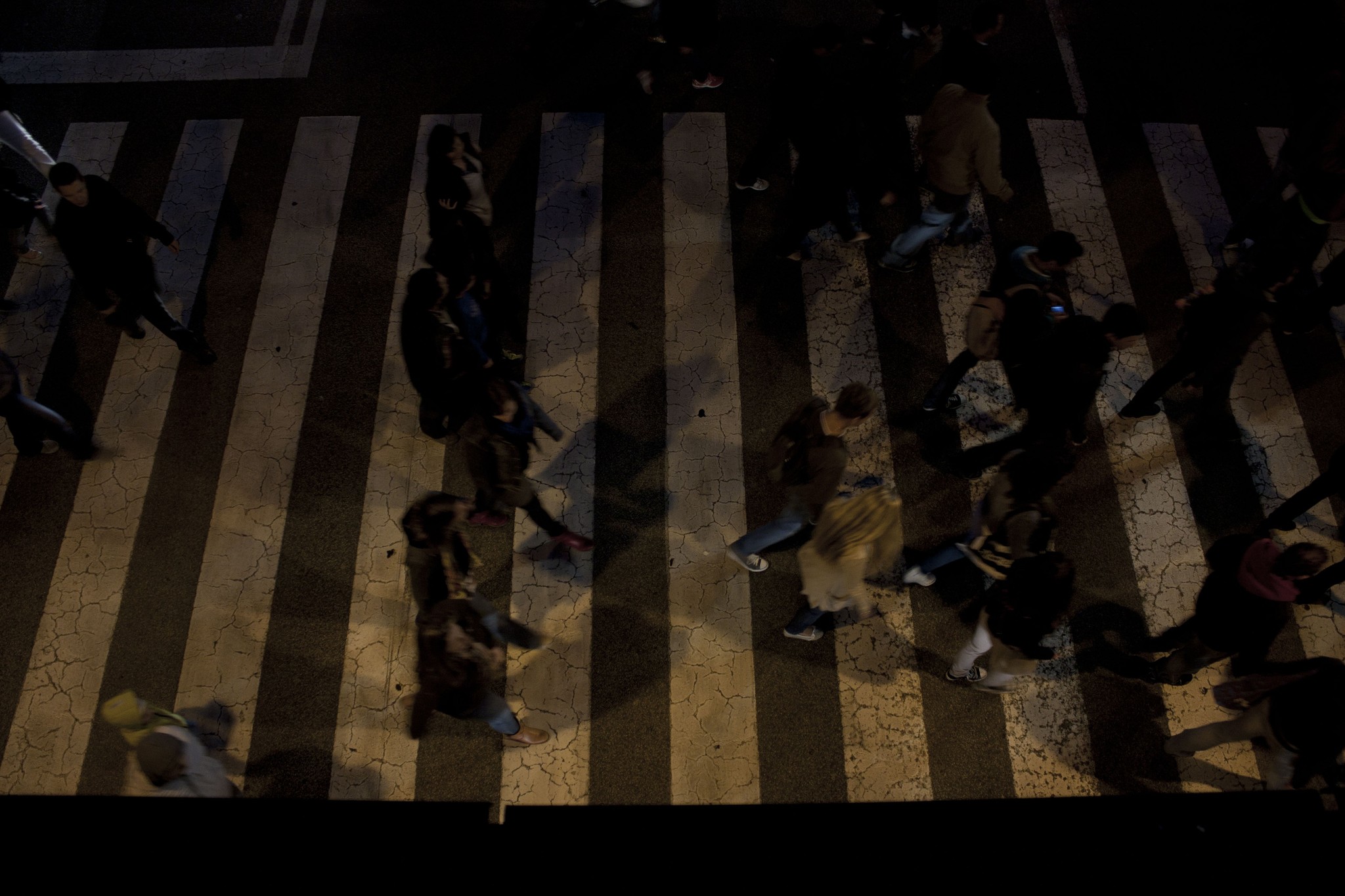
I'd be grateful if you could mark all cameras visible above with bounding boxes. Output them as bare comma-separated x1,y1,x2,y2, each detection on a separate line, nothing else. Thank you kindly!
1051,305,1065,313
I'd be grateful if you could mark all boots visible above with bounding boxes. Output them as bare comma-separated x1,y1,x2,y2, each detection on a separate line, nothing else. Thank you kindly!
469,507,509,527
550,524,596,552
504,713,550,744
398,694,418,711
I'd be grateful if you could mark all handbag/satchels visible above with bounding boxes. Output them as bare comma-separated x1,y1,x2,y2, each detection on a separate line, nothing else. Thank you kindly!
1213,667,1318,709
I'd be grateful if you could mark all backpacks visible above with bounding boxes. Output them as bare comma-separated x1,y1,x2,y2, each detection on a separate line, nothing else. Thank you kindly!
965,283,1038,361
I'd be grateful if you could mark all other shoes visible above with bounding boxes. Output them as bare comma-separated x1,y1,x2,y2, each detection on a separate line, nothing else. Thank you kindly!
1121,403,1161,419
977,681,1016,694
1267,520,1296,532
194,347,218,363
736,177,770,190
1163,735,1195,757
1142,661,1193,686
40,440,60,454
877,257,917,272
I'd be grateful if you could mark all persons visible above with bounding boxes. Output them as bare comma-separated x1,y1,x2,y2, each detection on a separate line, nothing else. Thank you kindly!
49,161,216,364
724,382,905,641
0,348,119,467
619,0,725,97
399,122,599,748
862,0,1345,787
99,688,241,795
0,171,55,261
732,39,798,190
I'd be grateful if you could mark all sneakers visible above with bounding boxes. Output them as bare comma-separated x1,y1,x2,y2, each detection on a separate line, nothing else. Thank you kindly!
17,248,44,261
725,541,769,572
783,625,823,641
692,72,724,88
946,663,988,681
903,565,936,586
923,393,968,410
